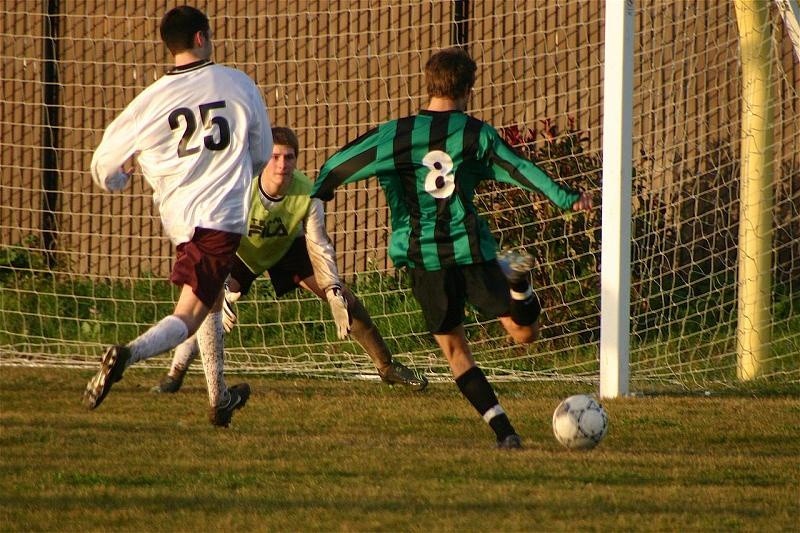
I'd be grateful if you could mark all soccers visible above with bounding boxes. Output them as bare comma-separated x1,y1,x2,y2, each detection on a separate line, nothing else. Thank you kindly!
551,394,610,450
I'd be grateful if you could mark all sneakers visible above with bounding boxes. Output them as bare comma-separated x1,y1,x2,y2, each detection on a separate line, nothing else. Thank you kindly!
149,377,180,396
495,244,535,285
492,436,520,452
377,361,428,391
82,346,131,412
210,382,251,427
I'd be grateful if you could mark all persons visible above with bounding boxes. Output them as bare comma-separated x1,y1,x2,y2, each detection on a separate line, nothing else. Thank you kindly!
151,124,431,392
79,0,275,429
315,51,594,445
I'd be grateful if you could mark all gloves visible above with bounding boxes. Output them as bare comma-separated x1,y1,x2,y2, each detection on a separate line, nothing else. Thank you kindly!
220,286,242,335
326,290,353,342
